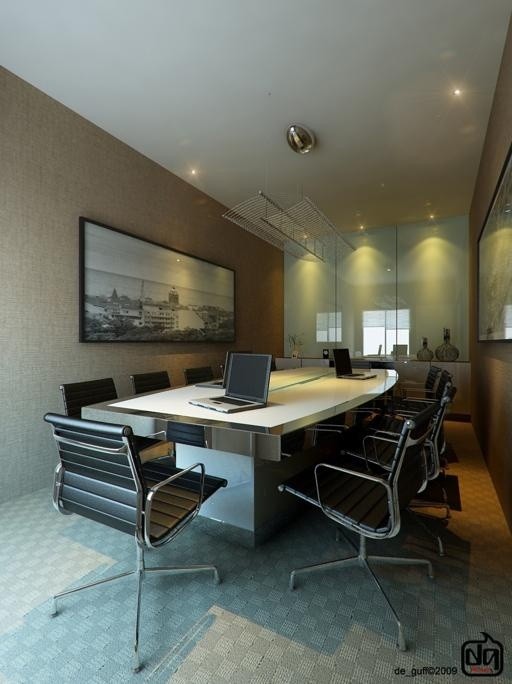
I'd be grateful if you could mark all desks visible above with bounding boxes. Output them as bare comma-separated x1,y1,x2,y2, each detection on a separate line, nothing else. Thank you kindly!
80,365,400,550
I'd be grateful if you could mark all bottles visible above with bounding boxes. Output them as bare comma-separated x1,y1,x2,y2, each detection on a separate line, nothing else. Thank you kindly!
434,328,459,361
417,337,433,360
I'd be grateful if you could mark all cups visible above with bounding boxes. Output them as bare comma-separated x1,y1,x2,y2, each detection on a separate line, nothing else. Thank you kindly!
321,348,329,358
328,358,334,367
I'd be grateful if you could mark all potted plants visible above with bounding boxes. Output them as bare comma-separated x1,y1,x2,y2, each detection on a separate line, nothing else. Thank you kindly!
287,332,306,358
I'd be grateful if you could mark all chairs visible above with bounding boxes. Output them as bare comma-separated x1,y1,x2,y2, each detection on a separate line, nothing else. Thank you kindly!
43,411,228,675
129,369,172,395
59,377,118,420
184,365,214,385
276,356,459,653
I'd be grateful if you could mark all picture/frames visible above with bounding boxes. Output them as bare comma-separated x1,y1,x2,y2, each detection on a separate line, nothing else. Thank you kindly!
78,215,236,341
477,140,512,341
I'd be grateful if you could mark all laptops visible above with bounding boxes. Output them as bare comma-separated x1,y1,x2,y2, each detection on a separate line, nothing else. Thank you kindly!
188,351,274,415
194,350,231,389
333,348,377,380
